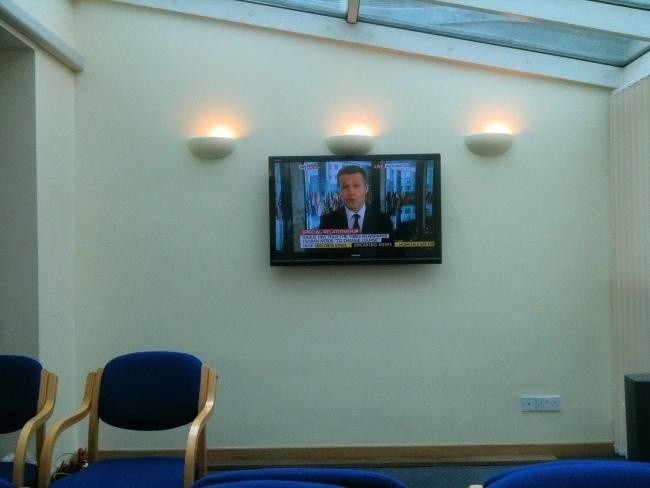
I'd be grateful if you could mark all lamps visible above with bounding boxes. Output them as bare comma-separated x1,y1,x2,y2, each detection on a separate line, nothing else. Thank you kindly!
326,133,375,155
187,135,235,159
465,133,513,156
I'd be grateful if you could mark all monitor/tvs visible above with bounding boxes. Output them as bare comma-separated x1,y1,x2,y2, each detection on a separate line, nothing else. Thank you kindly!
268,153,442,266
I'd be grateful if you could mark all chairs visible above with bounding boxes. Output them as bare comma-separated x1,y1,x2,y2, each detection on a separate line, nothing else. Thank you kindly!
42,351,219,485
198,459,650,486
0,351,60,487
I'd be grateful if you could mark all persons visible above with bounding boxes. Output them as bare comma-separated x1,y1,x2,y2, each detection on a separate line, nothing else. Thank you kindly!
314,165,393,234
